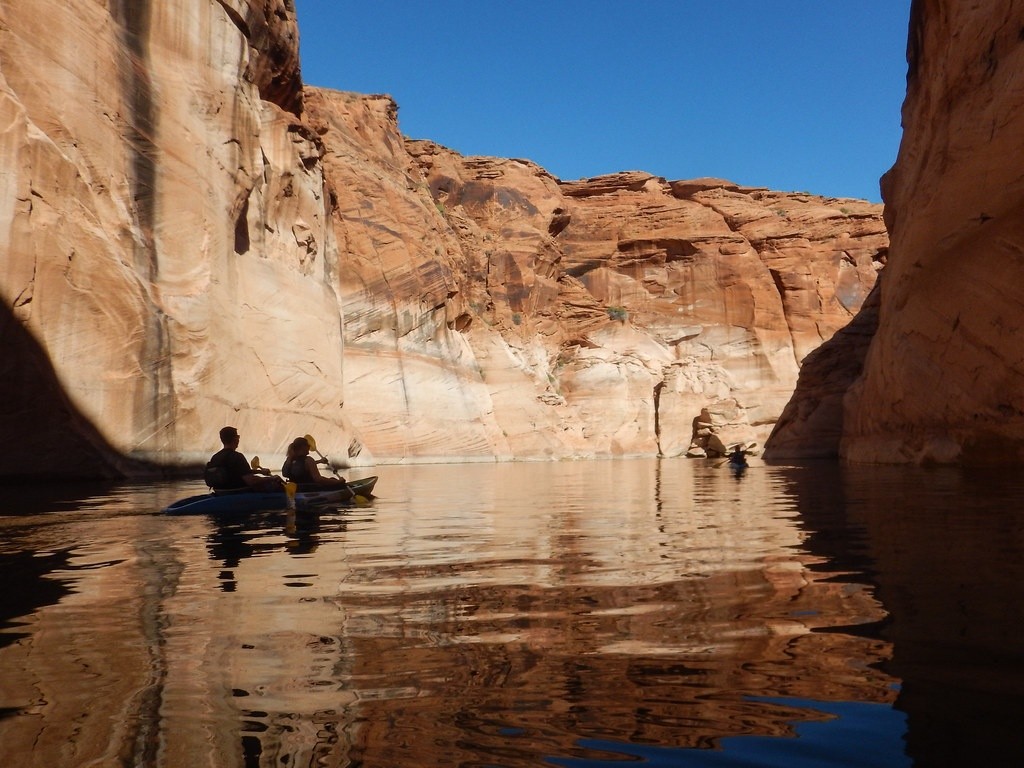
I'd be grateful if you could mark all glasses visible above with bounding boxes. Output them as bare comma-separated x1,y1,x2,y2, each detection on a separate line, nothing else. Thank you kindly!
236,435,241,439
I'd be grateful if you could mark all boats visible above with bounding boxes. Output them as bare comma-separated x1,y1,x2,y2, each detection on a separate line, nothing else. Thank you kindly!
158,475,379,516
729,458,749,471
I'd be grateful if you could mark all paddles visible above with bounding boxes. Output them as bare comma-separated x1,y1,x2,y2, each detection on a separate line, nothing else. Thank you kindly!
305,433,370,502
712,443,758,467
250,456,296,495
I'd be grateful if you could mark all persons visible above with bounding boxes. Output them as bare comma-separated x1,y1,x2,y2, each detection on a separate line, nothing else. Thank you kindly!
282,437,346,490
730,445,747,464
206,426,281,495
